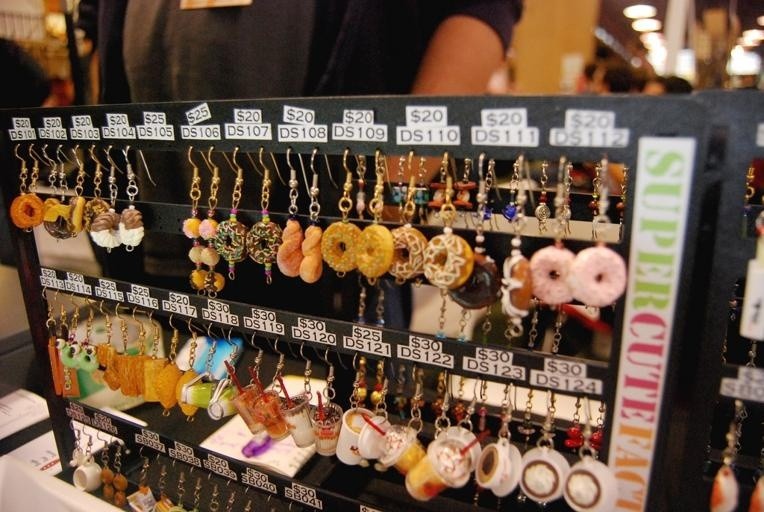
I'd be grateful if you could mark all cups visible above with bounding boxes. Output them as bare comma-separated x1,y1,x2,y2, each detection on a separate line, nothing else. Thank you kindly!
517,447,564,502
563,458,610,511
358,417,393,461
307,402,341,457
336,407,373,468
72,455,103,492
476,444,511,490
178,371,239,422
404,428,471,502
227,385,314,450
378,424,426,476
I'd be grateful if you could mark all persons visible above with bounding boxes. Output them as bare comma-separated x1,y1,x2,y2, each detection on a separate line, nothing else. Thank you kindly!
69,0,149,287
0,38,52,266
121,0,526,385
577,62,694,99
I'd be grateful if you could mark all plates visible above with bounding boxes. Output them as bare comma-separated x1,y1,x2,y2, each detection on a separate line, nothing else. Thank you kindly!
491,446,522,497
522,446,572,480
572,459,618,512
